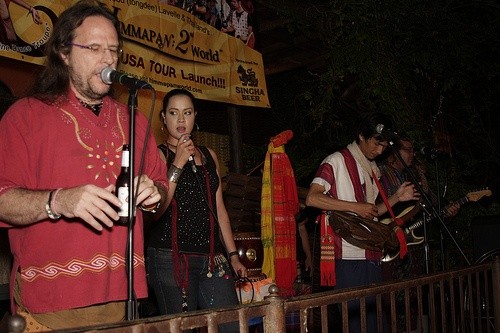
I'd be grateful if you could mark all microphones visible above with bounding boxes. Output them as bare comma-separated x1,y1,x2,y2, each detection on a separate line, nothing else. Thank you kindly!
375,123,395,147
101,67,152,90
177,132,198,174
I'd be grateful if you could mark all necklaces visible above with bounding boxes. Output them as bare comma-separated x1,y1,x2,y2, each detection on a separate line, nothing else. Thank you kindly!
166,141,177,148
75,95,104,112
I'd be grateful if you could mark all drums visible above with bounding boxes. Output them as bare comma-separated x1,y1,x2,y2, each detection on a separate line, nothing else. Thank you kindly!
233,237,263,272
394,205,420,221
329,210,390,251
380,218,396,228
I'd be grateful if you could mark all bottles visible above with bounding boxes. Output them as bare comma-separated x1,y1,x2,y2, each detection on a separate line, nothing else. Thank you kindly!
114,144,136,226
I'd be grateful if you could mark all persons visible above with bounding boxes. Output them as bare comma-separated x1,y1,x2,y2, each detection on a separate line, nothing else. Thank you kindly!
0,2,169,333
195,0,254,44
146,86,249,333
377,132,461,269
305,110,424,333
0,0,42,47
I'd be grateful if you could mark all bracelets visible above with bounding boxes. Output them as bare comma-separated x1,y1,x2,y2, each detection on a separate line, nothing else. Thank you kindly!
46,187,65,220
166,162,185,184
137,202,161,215
227,251,239,258
50,187,64,217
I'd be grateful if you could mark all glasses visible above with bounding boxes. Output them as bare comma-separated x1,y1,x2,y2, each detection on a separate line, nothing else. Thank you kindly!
66,42,122,58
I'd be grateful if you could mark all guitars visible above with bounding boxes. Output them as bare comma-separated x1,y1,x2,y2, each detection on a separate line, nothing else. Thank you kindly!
381,187,492,263
